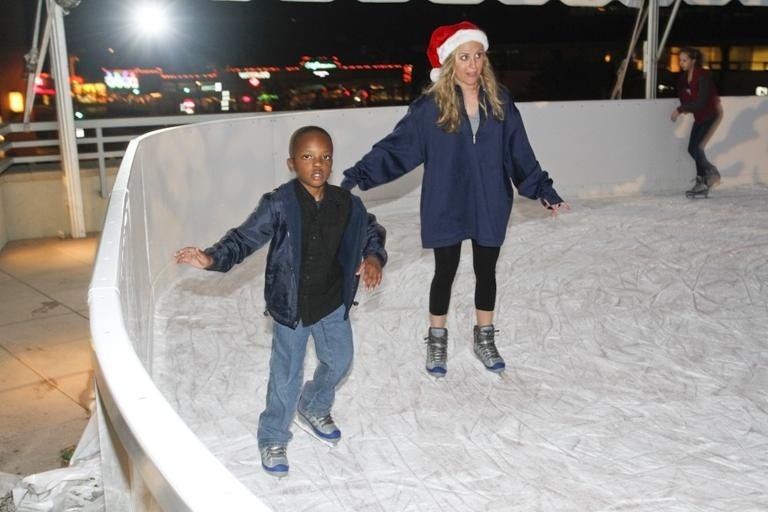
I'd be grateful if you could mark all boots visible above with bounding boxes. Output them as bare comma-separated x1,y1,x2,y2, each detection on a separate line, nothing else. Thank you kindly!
685,176,708,195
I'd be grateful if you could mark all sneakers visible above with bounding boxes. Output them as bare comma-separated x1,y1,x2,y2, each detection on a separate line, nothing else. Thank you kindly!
473,325,505,373
297,403,340,443
260,446,288,477
423,327,447,378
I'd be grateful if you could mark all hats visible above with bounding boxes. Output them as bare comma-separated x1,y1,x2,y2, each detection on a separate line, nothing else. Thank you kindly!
426,21,489,83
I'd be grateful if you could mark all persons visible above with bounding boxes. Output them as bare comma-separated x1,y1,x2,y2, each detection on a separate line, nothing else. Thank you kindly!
172,125,388,477
668,48,722,199
340,20,571,374
